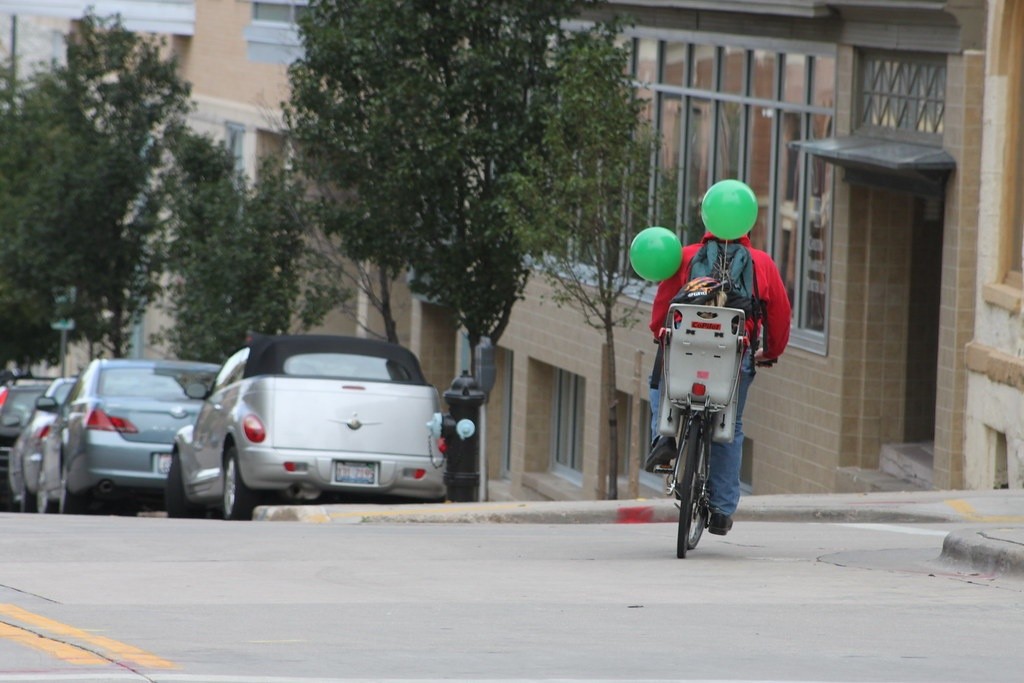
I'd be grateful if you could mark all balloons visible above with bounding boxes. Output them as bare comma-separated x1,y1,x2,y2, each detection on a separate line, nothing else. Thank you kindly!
701,179,759,241
629,225,682,283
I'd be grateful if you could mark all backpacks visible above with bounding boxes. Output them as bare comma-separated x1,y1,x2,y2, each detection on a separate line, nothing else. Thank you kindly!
689,242,754,318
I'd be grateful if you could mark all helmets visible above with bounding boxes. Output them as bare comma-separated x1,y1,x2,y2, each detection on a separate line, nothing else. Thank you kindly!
670,278,730,304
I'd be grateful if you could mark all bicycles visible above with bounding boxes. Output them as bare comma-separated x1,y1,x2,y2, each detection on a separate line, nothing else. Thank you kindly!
653,302,748,560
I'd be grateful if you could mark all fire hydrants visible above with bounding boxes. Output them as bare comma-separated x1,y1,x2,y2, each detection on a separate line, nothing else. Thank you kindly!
427,369,486,502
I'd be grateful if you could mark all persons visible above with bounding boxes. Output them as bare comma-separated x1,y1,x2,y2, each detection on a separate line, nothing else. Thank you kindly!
644,228,792,536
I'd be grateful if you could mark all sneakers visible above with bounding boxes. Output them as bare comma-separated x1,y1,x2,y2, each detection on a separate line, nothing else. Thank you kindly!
709,512,735,536
644,436,677,473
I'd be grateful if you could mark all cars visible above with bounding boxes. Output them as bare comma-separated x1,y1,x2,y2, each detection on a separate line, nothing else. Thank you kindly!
164,331,447,520
35,357,223,516
0,376,75,514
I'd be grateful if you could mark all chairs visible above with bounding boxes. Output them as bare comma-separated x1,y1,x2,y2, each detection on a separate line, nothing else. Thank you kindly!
658,302,745,444
